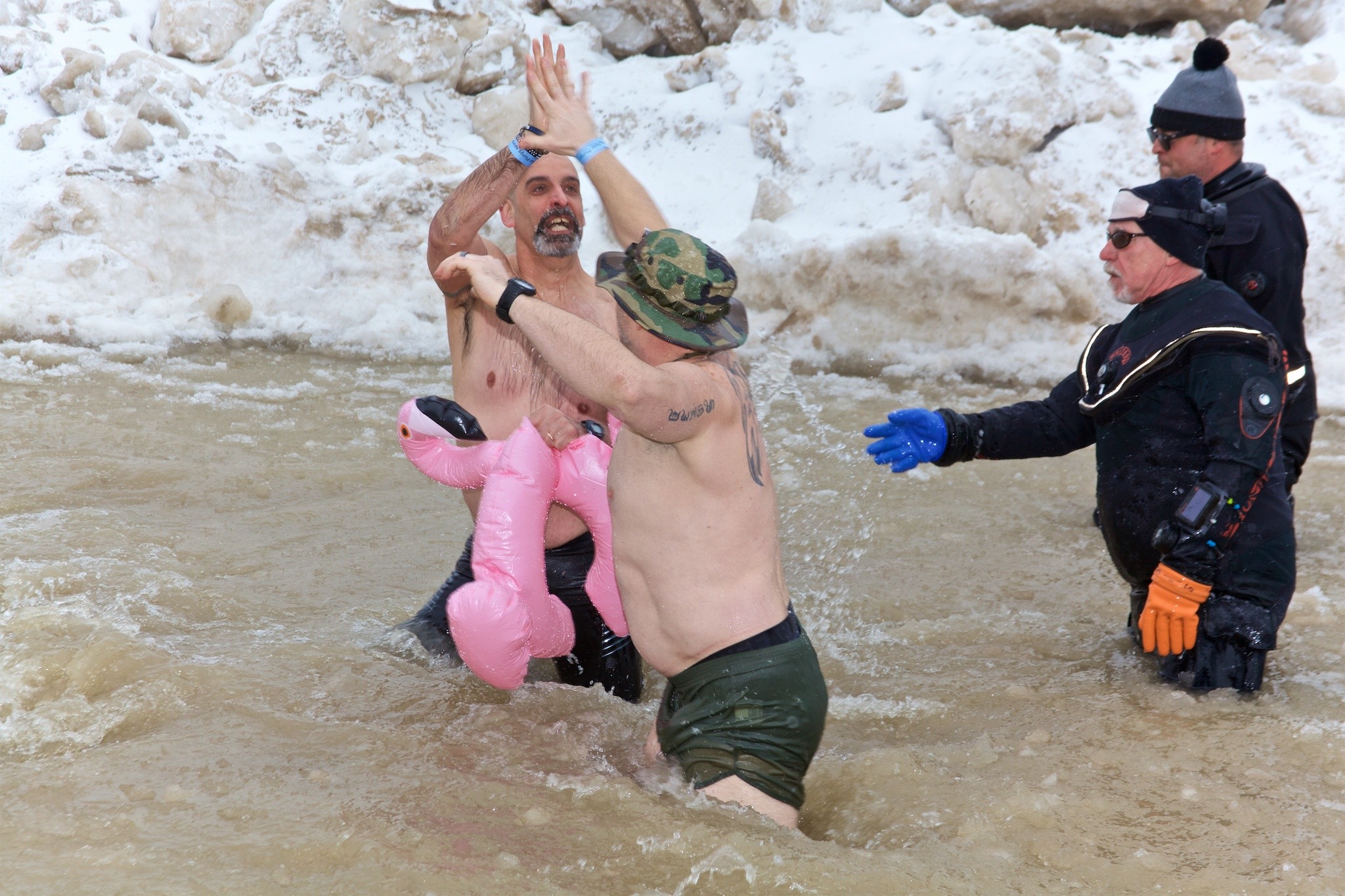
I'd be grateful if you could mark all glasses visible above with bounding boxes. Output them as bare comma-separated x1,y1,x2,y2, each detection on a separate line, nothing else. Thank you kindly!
1106,232,1147,249
1147,124,1190,151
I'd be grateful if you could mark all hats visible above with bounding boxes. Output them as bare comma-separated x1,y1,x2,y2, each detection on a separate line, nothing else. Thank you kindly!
595,229,748,354
1130,174,1209,269
1149,38,1246,140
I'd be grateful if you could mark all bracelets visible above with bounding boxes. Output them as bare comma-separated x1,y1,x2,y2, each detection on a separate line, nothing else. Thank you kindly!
520,124,549,153
508,134,542,168
575,136,607,164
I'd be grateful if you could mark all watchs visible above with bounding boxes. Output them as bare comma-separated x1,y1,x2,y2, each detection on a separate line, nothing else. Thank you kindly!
496,278,536,324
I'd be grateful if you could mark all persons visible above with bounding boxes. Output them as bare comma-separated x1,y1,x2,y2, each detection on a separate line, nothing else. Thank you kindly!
1145,37,1320,487
391,30,644,707
861,175,1297,693
431,56,828,828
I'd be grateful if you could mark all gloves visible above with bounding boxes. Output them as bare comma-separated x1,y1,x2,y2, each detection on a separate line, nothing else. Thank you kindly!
863,409,950,473
1137,562,1212,657
